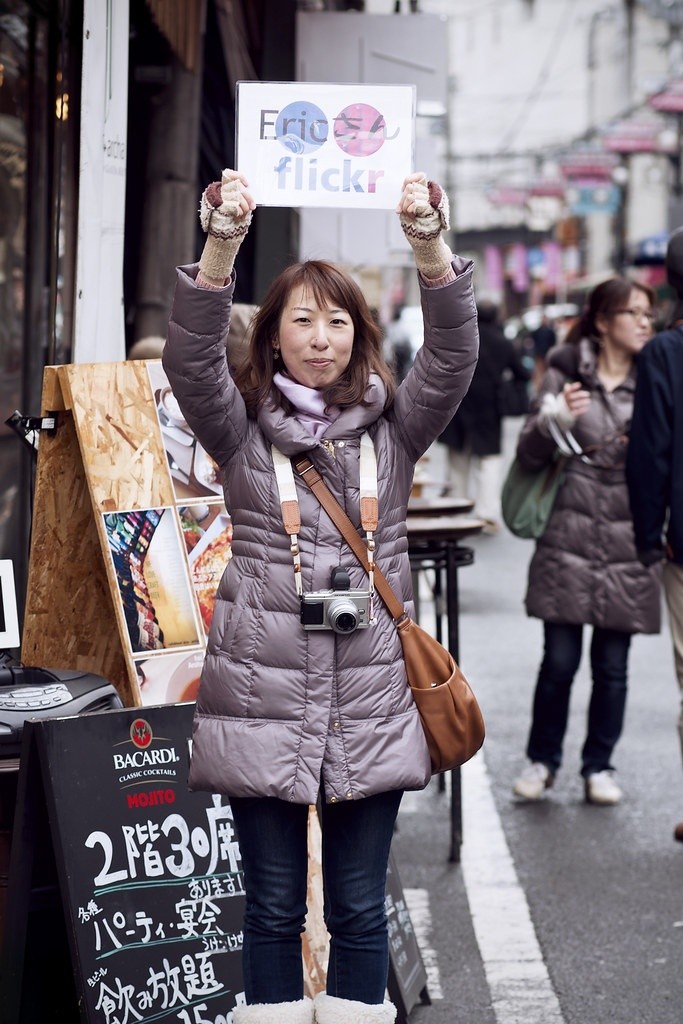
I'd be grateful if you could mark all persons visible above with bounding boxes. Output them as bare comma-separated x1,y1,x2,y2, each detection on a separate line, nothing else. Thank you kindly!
514,228,683,843
127,297,680,543
162,168,479,1024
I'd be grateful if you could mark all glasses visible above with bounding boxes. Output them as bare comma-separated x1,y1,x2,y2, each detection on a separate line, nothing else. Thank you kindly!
614,307,658,320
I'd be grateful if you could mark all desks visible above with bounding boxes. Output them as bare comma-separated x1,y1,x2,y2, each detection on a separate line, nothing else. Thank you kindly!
406,495,475,791
406,517,484,867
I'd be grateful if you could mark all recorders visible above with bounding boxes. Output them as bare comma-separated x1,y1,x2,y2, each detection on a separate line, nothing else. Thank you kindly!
0,666,124,760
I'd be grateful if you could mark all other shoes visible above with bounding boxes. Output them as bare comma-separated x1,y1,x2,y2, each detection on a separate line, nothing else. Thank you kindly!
674,823,683,842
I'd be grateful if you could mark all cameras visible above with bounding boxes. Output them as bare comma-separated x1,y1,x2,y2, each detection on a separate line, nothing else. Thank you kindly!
300,566,372,634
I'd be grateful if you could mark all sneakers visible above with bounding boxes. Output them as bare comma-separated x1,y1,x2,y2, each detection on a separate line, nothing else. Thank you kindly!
585,771,623,804
512,762,553,800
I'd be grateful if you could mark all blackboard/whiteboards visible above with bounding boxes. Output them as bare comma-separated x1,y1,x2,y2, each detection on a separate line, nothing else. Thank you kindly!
385,846,429,1020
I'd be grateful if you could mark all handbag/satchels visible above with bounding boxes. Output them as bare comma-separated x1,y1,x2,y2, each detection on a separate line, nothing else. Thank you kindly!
500,371,574,541
397,618,485,775
